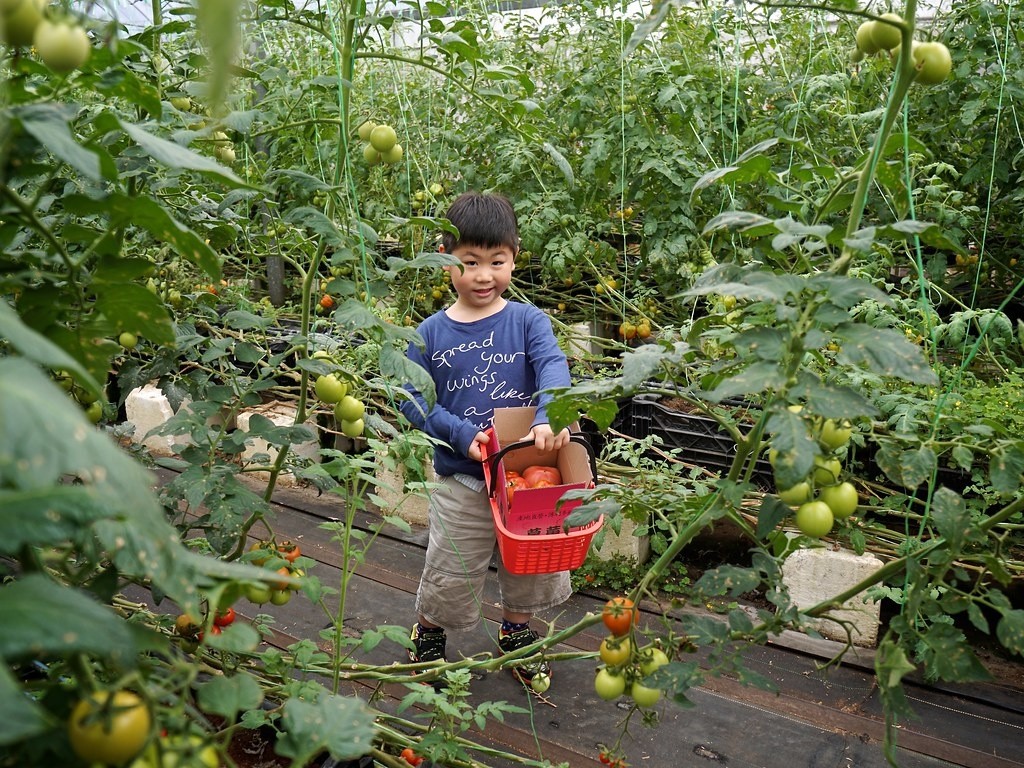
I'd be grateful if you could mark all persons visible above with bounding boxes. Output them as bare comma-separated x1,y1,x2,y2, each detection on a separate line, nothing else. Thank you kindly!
400,194,571,699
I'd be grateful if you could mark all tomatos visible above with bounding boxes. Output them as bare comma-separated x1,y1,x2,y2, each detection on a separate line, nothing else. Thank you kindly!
505,15,990,768
0,1,464,767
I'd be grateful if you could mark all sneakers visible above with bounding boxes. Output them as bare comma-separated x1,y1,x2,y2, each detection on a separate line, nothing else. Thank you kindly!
406,622,449,693
498,624,552,684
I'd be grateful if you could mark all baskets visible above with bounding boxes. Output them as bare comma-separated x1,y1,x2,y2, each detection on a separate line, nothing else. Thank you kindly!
478,426,604,575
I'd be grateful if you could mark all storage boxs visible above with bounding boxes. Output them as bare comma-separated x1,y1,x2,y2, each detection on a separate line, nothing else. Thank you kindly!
489,406,593,532
469,430,606,576
